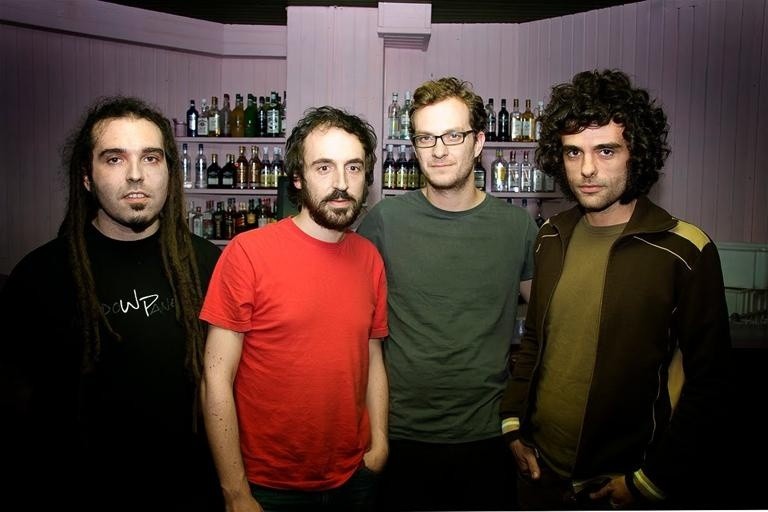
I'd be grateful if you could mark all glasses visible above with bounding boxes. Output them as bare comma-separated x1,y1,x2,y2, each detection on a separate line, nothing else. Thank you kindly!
410,129,476,149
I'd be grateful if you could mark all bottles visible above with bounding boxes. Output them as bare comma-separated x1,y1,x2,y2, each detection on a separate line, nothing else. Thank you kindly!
174,89,286,243
509,290,529,373
506,198,545,228
383,89,555,194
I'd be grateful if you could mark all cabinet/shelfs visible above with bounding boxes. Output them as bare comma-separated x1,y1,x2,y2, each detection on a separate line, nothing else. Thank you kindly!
379,137,562,201
168,138,282,245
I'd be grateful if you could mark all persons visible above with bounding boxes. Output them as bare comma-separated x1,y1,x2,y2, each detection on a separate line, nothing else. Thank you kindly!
199,104,391,512
0,94,223,512
353,74,539,511
496,65,734,509
